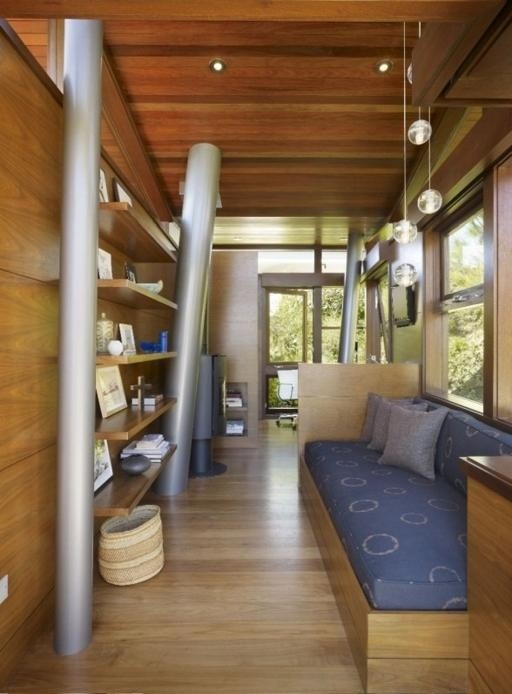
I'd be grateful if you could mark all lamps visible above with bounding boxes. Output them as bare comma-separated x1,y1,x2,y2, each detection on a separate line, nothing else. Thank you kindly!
393,22,443,287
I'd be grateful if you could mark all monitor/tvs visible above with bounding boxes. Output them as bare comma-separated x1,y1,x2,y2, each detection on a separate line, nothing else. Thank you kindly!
390,284,413,326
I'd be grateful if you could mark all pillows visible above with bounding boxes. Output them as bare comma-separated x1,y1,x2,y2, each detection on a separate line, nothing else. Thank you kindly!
367,396,427,454
377,405,449,481
359,393,415,443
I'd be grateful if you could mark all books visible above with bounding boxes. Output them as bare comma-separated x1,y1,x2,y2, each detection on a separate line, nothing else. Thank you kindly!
226,391,243,407
119,432,171,463
131,394,163,406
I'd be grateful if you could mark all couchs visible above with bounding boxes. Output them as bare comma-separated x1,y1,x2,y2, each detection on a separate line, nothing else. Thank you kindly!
297,393,512,694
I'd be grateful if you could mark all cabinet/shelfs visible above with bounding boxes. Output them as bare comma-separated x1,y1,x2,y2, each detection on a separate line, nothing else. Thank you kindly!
92,202,178,515
217,381,249,436
455,454,512,694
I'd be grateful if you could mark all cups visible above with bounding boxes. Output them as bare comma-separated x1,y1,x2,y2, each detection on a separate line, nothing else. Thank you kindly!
138,342,162,354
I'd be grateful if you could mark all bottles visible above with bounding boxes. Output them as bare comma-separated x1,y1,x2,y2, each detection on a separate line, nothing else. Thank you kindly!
122,454,151,476
96,309,122,357
158,328,171,353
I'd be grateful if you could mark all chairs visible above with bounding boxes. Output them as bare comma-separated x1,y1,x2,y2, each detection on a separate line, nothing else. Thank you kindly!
275,368,298,430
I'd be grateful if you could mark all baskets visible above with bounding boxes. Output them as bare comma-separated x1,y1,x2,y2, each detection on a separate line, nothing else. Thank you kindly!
96,504,164,585
